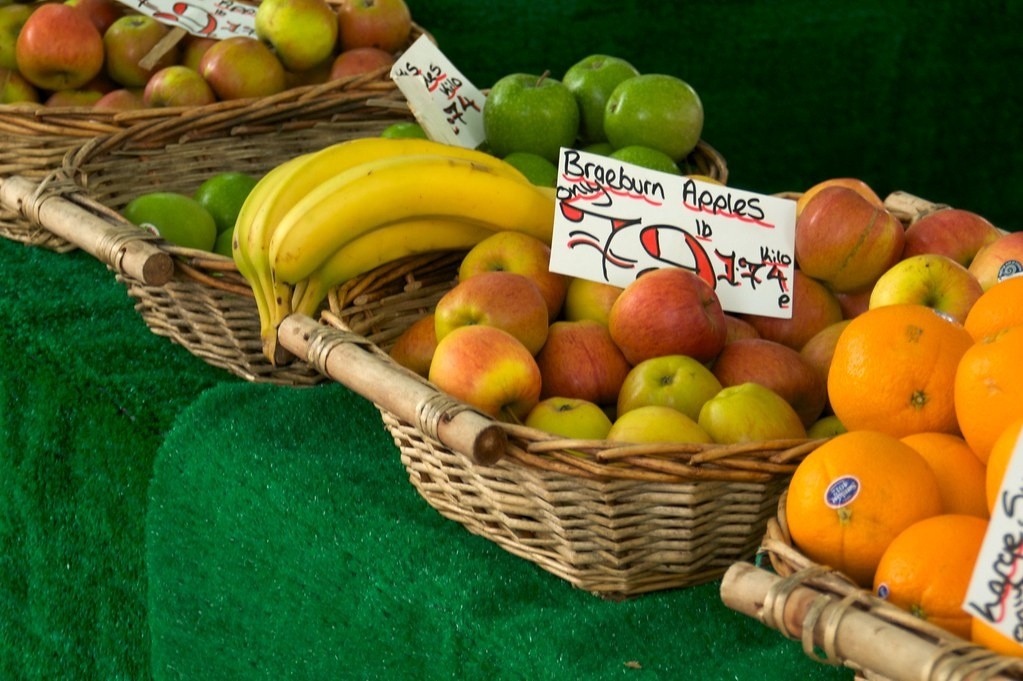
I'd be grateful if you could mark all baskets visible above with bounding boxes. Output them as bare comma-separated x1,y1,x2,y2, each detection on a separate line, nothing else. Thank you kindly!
718,485,1023,681
0,19,438,252
279,190,1012,596
0,95,729,387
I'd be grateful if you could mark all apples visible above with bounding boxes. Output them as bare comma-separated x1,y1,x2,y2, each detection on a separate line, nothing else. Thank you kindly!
0,0,1023,461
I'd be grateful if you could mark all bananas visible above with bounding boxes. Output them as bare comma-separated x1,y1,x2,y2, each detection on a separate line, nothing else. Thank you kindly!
232,135,556,370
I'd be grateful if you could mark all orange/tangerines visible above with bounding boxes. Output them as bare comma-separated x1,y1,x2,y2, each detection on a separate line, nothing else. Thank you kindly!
785,274,1023,661
